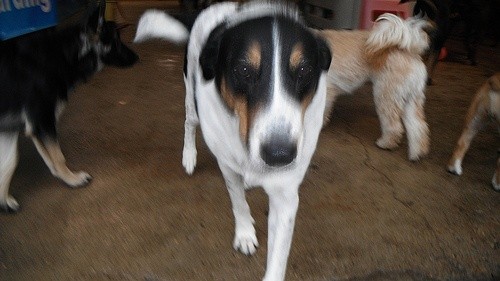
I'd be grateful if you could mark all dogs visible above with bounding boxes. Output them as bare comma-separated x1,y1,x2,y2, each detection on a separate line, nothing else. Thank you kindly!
132,0,332,281
397,0,500,190
305,8,431,163
0,0,140,213
